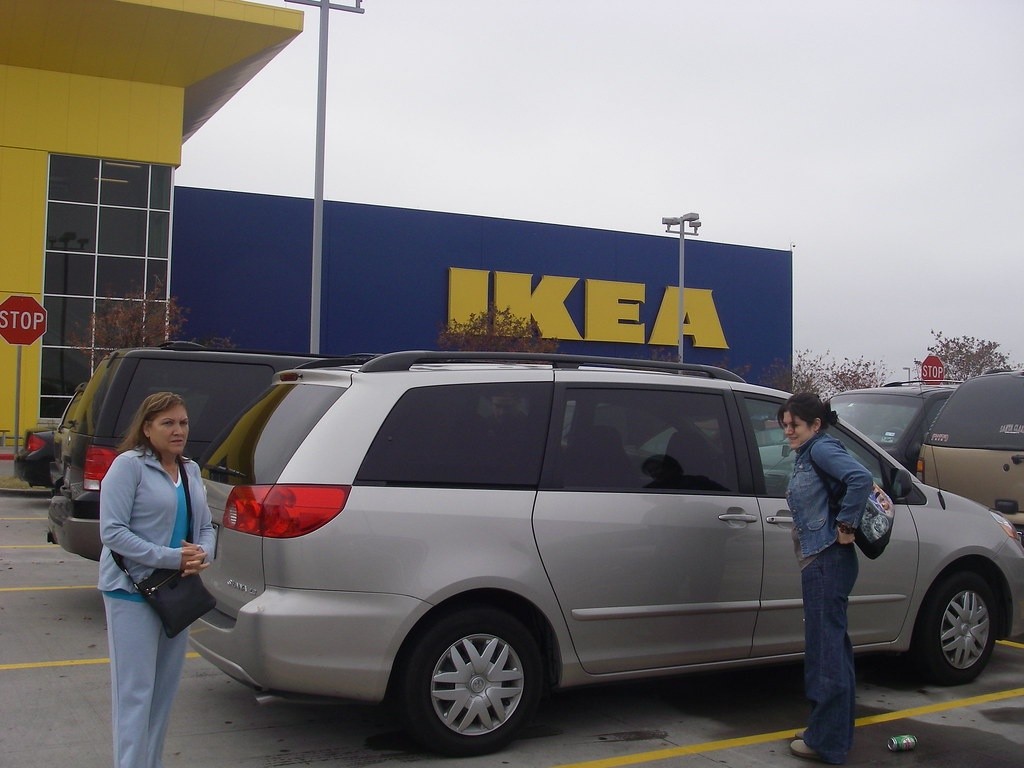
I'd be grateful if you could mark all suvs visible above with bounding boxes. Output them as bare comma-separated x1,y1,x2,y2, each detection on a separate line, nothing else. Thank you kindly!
47,341,345,562
186,353,1023,758
820,380,967,504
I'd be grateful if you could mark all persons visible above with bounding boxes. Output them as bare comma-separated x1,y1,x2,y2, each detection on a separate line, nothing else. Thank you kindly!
489,396,528,422
95,392,217,768
776,393,875,765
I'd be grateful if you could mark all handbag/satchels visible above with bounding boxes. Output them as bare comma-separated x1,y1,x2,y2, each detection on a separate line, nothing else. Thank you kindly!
134,567,216,638
808,437,897,560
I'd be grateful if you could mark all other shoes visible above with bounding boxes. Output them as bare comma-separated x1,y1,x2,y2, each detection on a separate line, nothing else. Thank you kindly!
790,739,843,764
795,732,854,753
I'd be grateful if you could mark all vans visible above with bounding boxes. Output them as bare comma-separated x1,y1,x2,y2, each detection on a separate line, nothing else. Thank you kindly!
914,371,1024,541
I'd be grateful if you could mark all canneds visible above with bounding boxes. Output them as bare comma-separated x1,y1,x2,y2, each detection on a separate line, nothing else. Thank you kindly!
888,735,918,751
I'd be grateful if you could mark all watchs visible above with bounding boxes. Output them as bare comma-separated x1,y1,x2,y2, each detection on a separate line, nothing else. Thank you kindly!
835,520,857,534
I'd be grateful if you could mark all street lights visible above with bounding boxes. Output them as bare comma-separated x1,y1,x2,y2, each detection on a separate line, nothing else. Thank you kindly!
662,212,701,376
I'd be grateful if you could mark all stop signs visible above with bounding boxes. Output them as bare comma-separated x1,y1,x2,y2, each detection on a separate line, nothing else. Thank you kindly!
0,296,48,346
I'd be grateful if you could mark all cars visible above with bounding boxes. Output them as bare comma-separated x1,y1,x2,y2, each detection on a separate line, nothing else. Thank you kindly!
15,427,70,496
752,426,797,483
